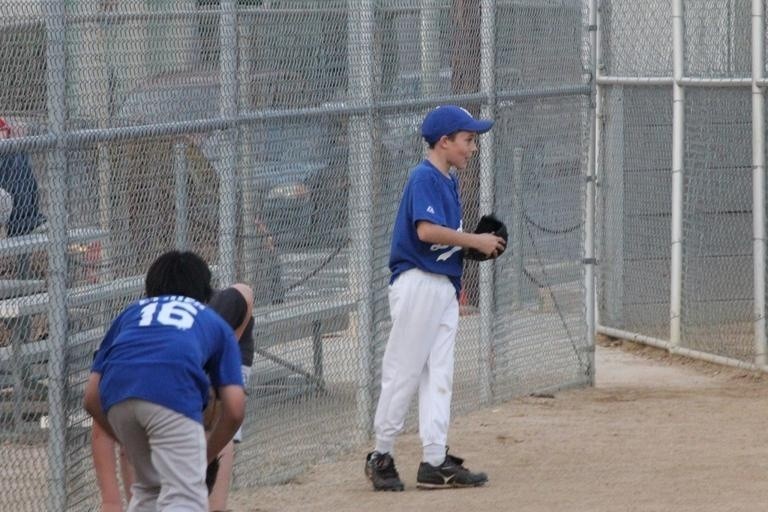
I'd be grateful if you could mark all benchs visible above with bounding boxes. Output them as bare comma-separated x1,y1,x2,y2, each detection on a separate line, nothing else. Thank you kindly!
1,224,359,446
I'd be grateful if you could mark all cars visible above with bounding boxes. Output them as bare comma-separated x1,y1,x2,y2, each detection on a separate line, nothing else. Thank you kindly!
1,105,281,425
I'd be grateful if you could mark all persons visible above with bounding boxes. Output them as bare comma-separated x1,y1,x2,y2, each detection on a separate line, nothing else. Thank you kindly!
79,247,249,512
88,280,257,512
0,112,45,242
361,102,509,494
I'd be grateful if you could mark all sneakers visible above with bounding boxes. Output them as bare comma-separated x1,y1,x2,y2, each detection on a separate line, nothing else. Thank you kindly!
363,451,407,493
416,460,489,488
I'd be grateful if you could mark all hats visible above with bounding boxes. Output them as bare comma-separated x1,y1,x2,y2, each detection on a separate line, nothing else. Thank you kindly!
420,105,494,145
